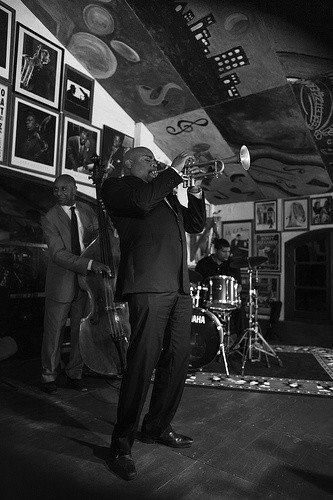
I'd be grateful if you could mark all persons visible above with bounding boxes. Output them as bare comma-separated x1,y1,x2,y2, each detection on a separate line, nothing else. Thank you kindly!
67,130,91,171
231,234,244,248
21,111,52,166
31,49,55,98
196,239,259,359
312,201,327,218
41,175,112,394
106,135,123,168
324,198,333,219
101,147,206,480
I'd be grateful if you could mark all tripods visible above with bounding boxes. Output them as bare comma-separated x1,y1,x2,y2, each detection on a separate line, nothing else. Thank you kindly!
223,264,284,378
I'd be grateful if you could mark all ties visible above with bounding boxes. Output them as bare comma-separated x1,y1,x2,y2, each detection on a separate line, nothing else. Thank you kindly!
70,207,81,257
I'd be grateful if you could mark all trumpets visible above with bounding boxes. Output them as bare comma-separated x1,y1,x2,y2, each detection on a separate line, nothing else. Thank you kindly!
152,145,251,188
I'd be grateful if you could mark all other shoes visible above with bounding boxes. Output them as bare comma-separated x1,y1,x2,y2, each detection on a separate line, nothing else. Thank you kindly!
69,378,89,391
42,381,58,395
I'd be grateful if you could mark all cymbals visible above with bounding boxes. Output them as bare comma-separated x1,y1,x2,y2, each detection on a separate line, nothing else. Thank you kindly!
188,269,202,282
230,256,267,268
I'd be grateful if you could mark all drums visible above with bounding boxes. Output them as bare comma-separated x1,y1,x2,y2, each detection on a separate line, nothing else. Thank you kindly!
200,275,240,309
191,307,224,371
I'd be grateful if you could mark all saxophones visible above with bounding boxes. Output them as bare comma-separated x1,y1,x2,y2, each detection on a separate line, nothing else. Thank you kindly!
19,43,41,87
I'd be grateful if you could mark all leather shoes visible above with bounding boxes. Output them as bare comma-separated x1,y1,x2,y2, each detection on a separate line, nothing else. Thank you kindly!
143,425,194,448
111,447,137,481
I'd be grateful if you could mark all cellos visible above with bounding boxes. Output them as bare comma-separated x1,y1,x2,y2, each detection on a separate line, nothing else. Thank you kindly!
77,152,132,377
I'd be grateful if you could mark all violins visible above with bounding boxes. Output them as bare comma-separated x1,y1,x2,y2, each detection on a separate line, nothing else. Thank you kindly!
33,130,49,153
